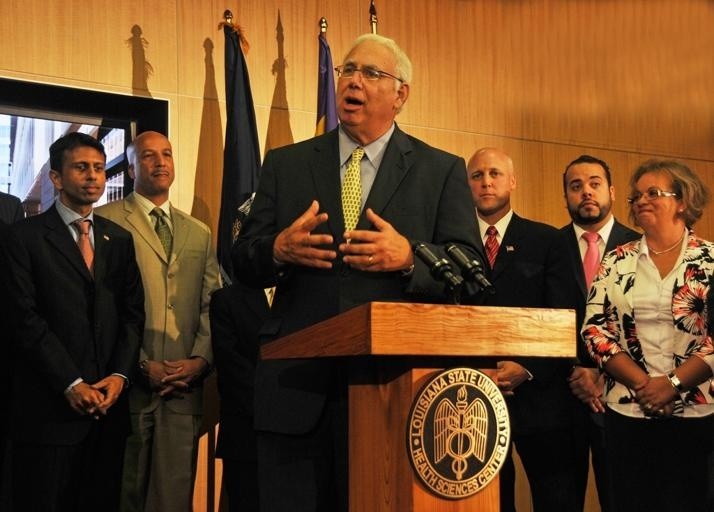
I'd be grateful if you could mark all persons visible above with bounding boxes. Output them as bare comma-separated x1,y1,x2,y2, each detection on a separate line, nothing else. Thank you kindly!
2,131,146,509
94,130,224,511
578,158,714,512
0,174,27,232
229,33,484,510
552,154,644,511
464,146,579,512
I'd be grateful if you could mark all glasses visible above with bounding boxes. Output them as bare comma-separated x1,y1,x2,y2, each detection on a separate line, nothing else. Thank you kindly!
335,64,403,83
627,188,677,204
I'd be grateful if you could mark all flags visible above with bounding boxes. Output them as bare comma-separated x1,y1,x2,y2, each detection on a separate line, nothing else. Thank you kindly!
315,18,338,139
216,20,263,289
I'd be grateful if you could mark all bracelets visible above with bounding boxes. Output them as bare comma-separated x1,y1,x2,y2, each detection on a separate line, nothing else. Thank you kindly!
139,359,148,369
668,372,684,393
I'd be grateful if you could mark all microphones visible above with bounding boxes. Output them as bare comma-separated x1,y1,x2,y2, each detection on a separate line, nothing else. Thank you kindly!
411,240,465,291
443,243,493,292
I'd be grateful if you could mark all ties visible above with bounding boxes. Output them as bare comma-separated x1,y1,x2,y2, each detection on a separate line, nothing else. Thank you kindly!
71,221,94,280
485,226,499,269
152,207,172,262
340,148,364,244
581,233,600,289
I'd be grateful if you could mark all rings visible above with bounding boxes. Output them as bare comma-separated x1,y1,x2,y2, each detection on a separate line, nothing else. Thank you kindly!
367,254,377,266
645,403,652,410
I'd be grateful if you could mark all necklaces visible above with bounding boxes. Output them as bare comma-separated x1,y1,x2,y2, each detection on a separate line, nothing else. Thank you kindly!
648,228,685,256
